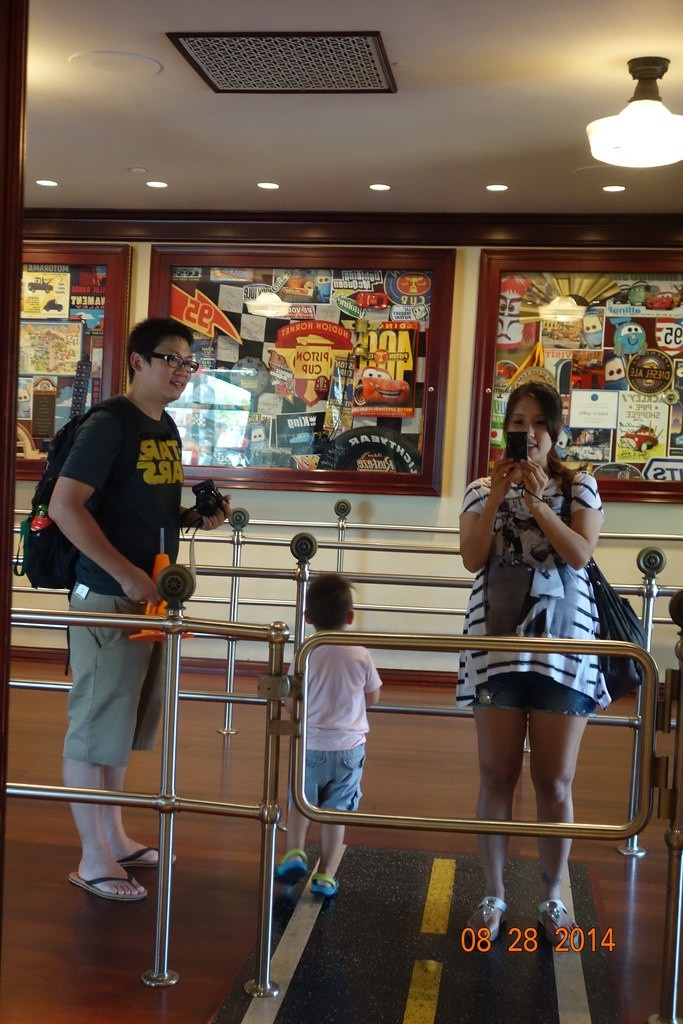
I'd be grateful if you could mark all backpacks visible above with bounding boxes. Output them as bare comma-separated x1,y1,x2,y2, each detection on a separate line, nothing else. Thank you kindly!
24,403,181,589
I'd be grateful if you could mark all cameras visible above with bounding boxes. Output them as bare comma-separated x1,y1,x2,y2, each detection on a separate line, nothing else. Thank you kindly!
192,479,230,518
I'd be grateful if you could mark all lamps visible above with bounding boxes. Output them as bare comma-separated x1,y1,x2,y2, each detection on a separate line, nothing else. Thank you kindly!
245,290,295,318
585,57,682,168
539,288,584,320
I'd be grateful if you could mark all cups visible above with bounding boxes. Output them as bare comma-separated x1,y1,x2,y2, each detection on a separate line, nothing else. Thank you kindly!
250,447,292,468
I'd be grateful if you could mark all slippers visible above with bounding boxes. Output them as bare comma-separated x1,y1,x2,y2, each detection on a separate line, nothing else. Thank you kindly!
537,898,581,948
466,896,509,944
68,871,148,901
115,845,175,868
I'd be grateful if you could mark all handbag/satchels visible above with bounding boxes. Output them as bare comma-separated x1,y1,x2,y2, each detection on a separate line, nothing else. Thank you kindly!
585,562,646,701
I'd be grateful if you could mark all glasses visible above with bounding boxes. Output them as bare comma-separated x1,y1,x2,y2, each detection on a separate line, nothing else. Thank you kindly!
149,352,199,374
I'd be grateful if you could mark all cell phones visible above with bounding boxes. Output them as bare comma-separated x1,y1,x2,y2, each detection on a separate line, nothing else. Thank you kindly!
505,431,528,464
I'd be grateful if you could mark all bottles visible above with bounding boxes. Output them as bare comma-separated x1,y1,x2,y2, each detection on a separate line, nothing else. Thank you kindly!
31,504,52,532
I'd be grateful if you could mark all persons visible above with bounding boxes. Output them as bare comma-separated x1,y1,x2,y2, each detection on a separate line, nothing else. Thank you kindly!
49,317,233,901
461,382,609,946
275,573,383,894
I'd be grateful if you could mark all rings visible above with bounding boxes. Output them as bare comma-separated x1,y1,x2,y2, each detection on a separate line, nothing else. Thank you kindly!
503,473,507,477
140,601,146,604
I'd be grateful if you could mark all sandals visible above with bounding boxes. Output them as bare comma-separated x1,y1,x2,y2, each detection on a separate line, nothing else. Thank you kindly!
311,872,340,895
275,850,310,884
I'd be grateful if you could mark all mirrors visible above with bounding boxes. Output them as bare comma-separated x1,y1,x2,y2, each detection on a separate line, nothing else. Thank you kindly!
0,0,683,1024
